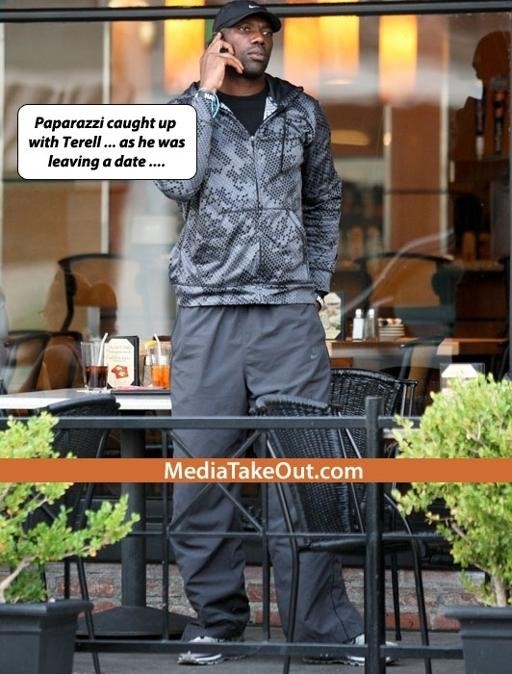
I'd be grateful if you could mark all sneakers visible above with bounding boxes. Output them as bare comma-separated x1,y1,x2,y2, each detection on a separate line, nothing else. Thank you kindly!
302,632,399,665
177,636,246,663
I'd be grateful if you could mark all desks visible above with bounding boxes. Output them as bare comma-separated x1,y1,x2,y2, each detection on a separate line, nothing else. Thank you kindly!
0,388,198,639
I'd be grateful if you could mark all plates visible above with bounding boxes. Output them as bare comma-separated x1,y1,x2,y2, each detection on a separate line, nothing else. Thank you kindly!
377,322,405,341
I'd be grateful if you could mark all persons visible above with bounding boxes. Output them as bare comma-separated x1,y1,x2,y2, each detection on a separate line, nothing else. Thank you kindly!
453,28,511,184
154,0,400,666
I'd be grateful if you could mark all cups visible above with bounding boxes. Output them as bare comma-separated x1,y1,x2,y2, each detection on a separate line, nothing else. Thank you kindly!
79,340,112,391
148,348,171,391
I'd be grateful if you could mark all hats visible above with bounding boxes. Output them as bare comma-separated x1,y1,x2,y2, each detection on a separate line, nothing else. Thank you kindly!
213,0,282,35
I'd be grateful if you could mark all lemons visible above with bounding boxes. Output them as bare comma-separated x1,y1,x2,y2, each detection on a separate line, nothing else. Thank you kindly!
145,340,156,350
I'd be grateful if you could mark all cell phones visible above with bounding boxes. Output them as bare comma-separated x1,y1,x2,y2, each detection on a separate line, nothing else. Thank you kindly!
206,34,232,73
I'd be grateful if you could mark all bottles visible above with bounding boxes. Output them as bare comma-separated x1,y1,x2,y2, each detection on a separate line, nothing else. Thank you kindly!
351,308,365,340
141,355,153,387
365,308,377,338
